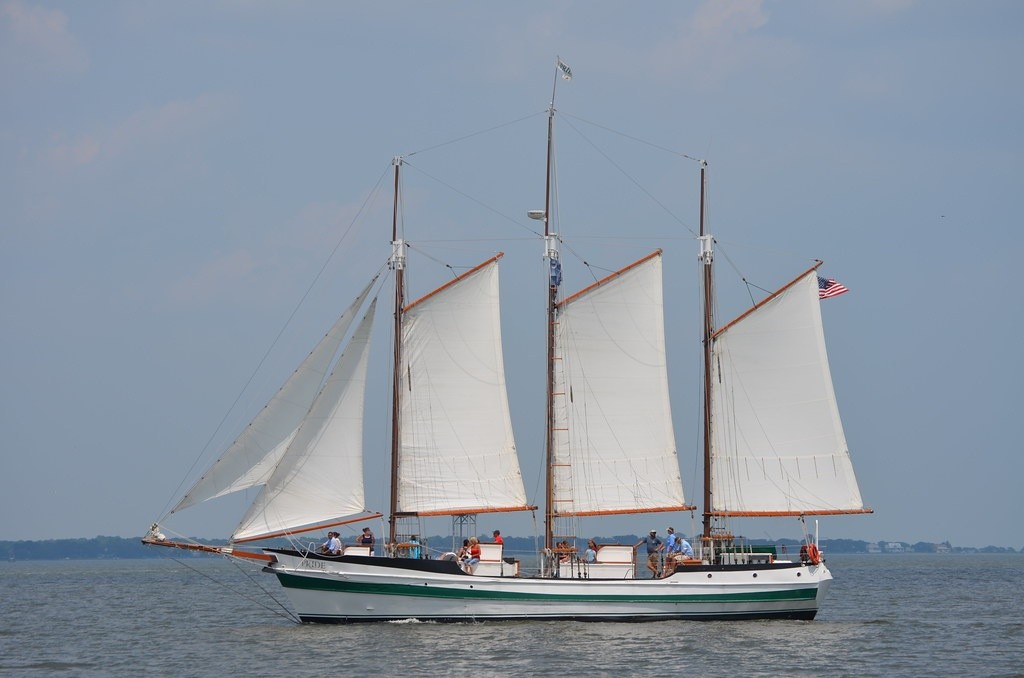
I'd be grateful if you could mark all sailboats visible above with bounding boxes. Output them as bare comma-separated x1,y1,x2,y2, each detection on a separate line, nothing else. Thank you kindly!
139,105,874,623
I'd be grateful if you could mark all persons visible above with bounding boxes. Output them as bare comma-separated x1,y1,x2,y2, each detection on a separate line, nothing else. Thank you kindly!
493,530,504,548
634,530,664,578
582,538,597,563
321,532,341,554
407,535,420,559
660,527,693,578
553,541,571,577
356,528,375,556
457,537,481,575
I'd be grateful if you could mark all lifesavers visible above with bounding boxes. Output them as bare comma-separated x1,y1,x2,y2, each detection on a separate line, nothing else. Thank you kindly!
800,544,819,565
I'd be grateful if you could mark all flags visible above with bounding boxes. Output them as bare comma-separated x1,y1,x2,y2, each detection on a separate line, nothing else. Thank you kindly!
818,277,848,299
